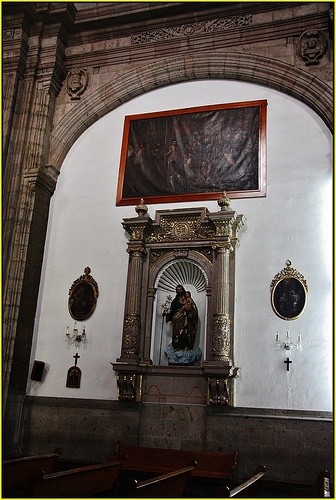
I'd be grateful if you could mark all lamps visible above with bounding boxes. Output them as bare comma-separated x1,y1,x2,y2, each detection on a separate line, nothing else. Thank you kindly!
65,322,86,350
275,329,302,349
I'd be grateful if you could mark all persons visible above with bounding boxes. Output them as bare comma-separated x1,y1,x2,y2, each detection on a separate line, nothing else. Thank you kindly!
179,291,196,316
165,285,199,352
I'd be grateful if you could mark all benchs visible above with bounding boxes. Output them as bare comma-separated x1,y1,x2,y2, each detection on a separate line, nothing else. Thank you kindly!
2,441,334,498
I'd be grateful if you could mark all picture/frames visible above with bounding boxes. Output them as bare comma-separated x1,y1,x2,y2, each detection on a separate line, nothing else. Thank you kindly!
270,260,308,320
68,267,99,321
